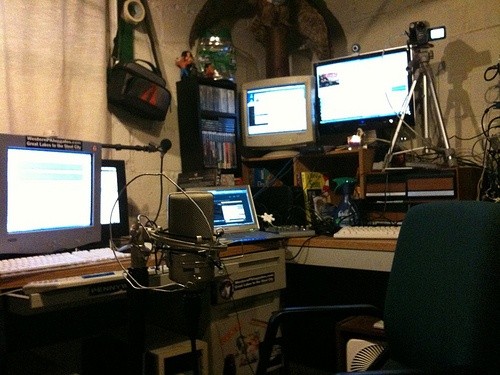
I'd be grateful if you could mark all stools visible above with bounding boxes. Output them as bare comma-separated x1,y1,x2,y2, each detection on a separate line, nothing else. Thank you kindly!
146,336,208,375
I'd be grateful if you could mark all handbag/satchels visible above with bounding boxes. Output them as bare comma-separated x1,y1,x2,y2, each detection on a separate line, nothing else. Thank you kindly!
107,58,171,121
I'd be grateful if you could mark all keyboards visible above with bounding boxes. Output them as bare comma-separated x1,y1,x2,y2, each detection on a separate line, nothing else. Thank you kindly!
334,225,400,241
23,264,168,293
0,246,131,278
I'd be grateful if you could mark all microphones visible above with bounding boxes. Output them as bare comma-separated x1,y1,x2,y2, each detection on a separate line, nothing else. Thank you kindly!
160,138,172,157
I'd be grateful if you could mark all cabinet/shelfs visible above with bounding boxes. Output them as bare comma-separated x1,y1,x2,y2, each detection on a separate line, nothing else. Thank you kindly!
177,78,243,181
244,145,460,272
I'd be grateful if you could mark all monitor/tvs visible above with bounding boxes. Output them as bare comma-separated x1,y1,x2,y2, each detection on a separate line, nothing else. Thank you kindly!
312,44,419,127
240,76,316,148
183,183,261,234
98,159,130,239
0,134,104,255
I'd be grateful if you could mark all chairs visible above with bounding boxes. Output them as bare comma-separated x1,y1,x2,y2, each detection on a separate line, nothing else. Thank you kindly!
339,202,500,375
255,304,382,375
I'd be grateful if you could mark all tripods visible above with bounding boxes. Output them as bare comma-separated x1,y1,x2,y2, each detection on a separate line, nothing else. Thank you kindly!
378,41,454,167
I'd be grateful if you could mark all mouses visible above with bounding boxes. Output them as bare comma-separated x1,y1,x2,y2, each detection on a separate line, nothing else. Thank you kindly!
118,245,132,253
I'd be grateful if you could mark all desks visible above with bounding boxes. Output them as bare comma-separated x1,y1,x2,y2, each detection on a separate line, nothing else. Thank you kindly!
0,281,213,375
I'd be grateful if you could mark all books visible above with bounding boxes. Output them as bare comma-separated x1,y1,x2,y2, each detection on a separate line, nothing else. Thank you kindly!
200,84,238,168
